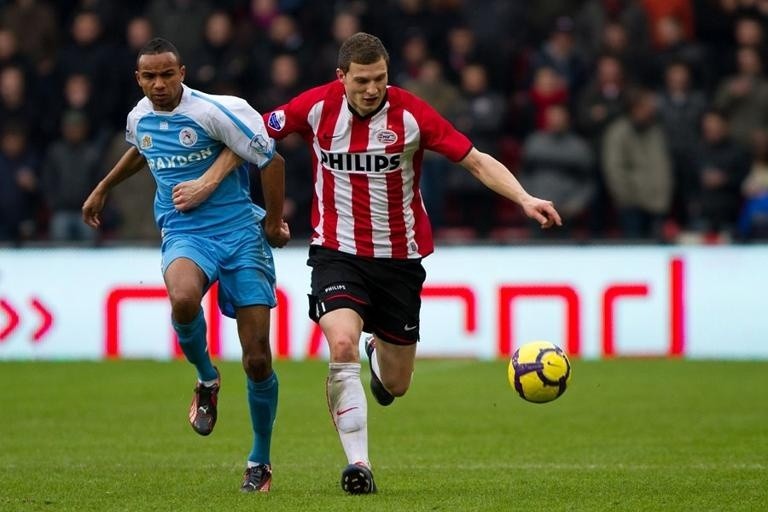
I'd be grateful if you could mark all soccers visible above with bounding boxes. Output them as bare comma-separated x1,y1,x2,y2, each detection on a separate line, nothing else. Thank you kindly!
508,342,570,403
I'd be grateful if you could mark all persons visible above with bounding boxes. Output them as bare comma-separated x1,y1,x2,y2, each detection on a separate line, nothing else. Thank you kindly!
82,37,291,493
0,0,768,248
171,32,563,494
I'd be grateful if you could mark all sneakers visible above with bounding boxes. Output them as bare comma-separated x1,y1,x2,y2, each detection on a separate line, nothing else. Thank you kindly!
364,336,395,406
190,366,220,436
240,463,271,493
341,462,377,495
